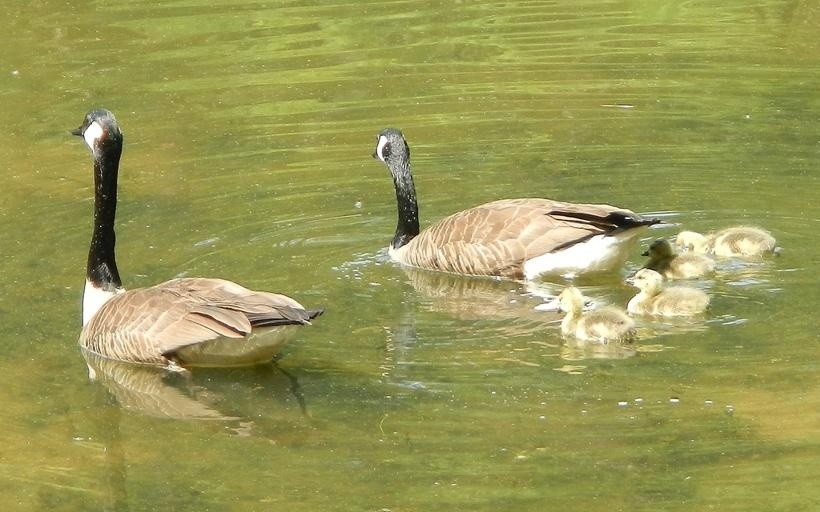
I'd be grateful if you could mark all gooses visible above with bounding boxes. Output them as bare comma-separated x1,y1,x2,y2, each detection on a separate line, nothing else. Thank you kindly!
371,127,664,281
532,221,776,347
66,105,325,374
